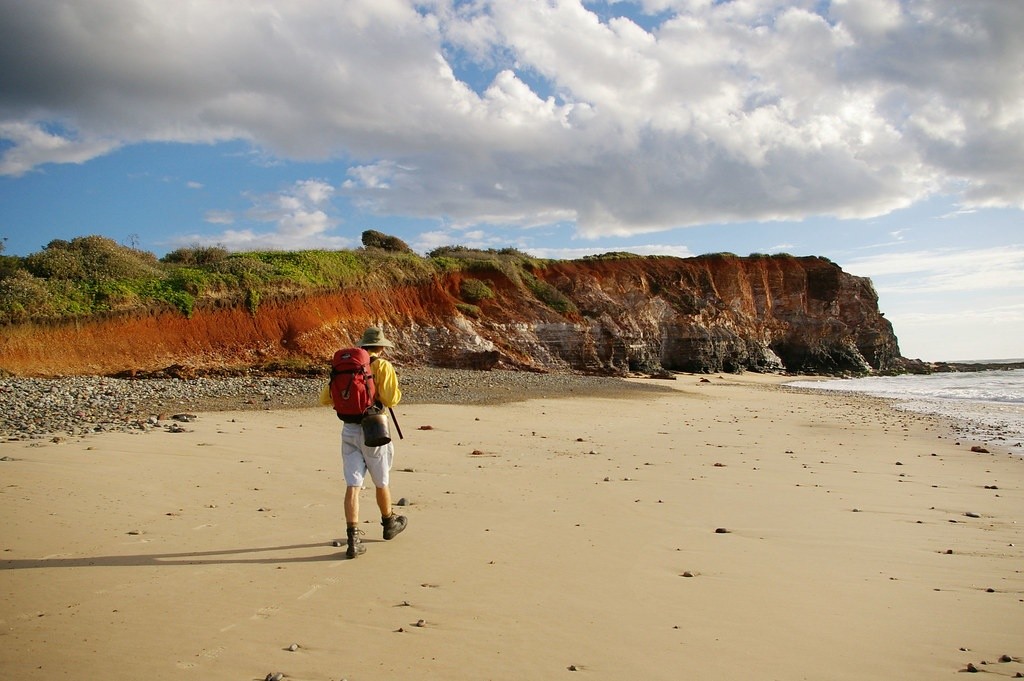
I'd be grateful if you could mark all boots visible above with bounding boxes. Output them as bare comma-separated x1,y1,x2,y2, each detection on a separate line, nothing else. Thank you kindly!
381,512,407,540
346,527,367,558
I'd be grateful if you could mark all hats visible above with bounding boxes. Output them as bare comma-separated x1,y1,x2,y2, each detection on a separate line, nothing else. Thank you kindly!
356,327,394,347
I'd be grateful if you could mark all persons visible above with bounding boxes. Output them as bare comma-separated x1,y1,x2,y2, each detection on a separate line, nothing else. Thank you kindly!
316,327,408,558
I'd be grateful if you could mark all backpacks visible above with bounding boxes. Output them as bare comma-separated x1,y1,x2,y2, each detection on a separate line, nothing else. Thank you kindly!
328,347,383,424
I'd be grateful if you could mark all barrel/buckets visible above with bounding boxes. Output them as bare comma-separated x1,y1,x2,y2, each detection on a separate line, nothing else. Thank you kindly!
361,406,392,447
